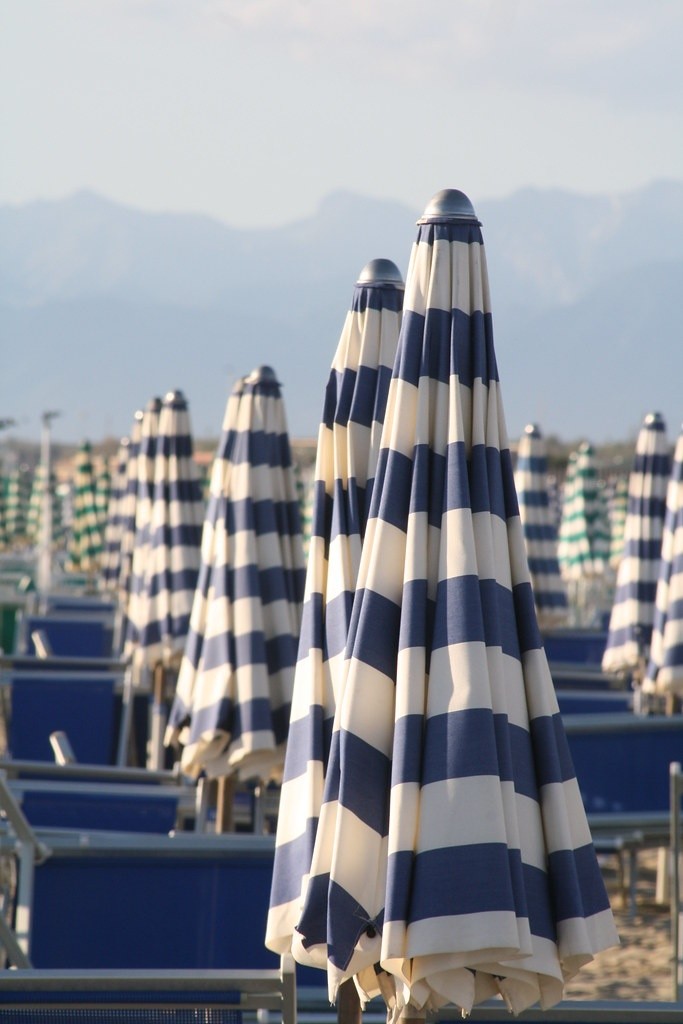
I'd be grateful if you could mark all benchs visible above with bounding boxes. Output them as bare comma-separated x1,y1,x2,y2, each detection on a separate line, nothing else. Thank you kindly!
0,594,683,1024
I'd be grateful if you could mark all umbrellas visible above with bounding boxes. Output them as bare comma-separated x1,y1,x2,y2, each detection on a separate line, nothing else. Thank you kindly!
601,412,673,712
562,443,628,629
296,188,618,1023
264,262,407,1023
517,423,568,625
645,424,683,713
0,365,307,838
